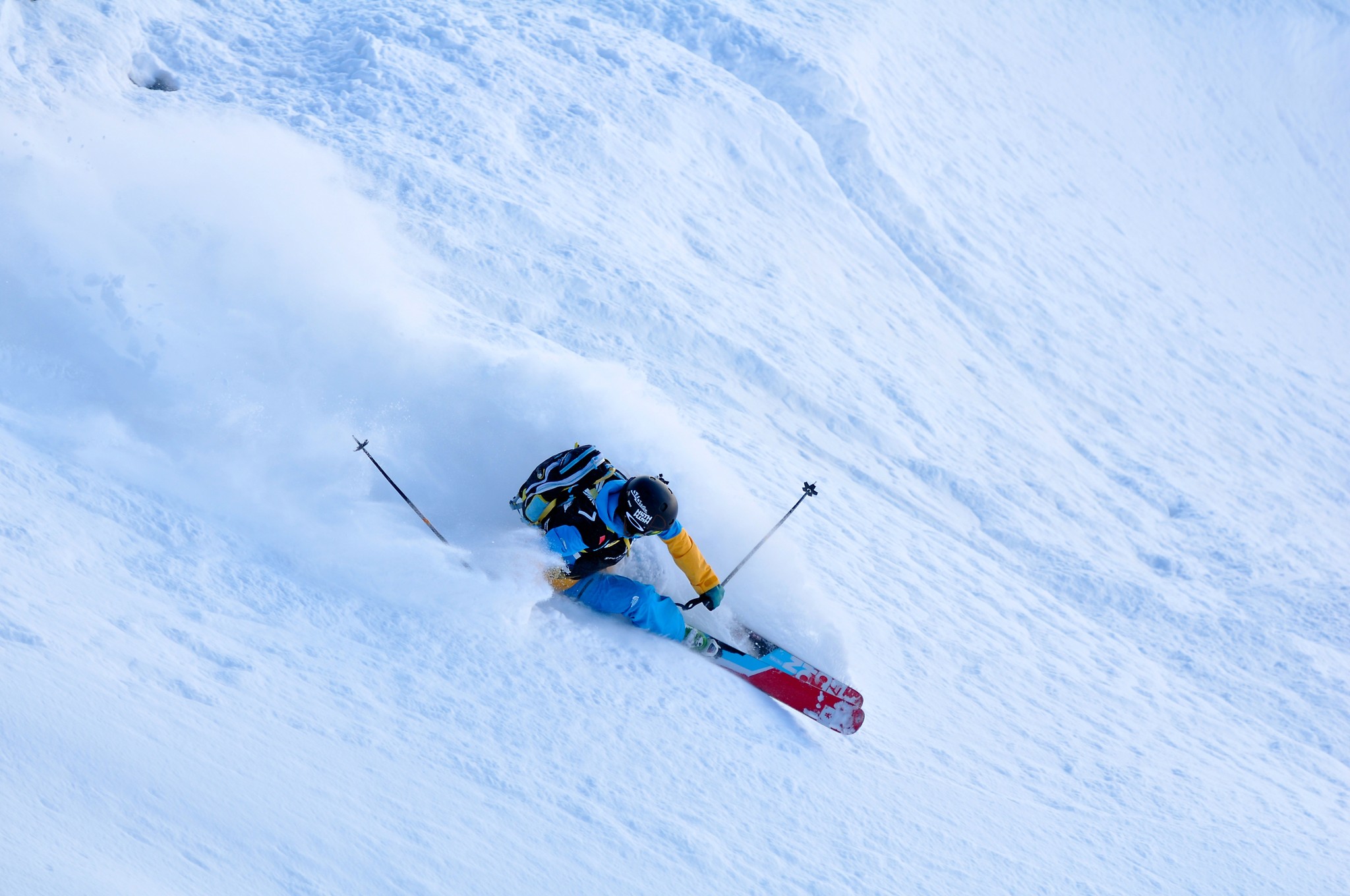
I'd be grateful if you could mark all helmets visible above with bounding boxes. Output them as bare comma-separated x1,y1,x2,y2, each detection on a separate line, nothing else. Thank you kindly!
620,475,679,538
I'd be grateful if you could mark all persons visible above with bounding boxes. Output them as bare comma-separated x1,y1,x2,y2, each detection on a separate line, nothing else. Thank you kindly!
508,442,723,640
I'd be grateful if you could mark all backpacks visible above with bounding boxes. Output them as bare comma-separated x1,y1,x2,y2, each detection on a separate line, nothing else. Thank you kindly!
509,444,626,549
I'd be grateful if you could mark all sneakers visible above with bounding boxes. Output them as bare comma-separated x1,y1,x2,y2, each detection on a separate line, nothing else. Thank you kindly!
683,624,706,651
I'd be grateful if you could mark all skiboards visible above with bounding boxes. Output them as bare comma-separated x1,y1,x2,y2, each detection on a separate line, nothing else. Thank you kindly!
682,598,866,735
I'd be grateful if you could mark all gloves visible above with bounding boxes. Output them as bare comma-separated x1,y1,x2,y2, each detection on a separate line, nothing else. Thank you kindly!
700,583,727,608
